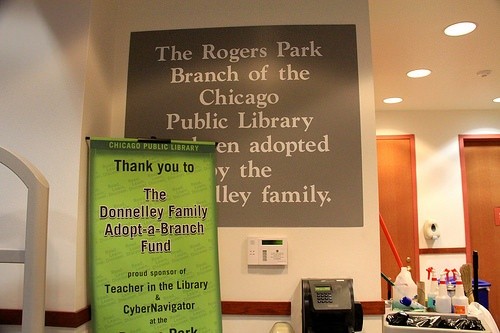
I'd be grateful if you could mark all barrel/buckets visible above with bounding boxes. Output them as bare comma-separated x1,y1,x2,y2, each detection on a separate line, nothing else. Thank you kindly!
438,275,491,312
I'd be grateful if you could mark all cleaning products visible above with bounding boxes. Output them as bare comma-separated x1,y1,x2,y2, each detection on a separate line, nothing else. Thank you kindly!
452,268,469,314
426,266,439,299
436,267,452,312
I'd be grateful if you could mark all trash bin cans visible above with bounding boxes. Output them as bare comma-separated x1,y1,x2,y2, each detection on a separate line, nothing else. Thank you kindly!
435,274,491,309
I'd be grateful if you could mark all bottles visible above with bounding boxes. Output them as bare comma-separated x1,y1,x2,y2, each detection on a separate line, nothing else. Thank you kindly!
435,268,451,313
393,267,418,303
426,267,438,312
451,269,468,315
